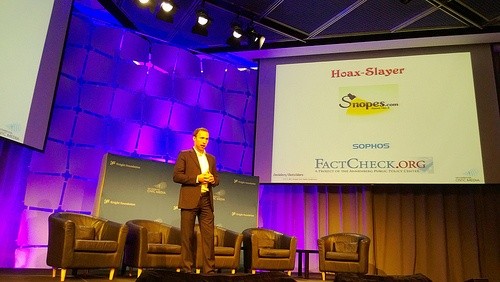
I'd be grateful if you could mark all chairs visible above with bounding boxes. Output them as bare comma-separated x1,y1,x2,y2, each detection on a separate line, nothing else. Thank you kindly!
242,228,296,276
46,212,129,282
194,224,244,274
317,233,371,280
121,220,182,277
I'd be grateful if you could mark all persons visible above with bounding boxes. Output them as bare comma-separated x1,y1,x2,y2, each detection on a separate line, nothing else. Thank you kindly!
174,128,220,274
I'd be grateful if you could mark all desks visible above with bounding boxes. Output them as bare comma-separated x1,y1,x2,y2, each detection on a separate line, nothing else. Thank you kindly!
296,250,319,277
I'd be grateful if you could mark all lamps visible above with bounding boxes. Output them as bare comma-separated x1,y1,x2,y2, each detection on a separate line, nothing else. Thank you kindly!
192,9,212,36
226,23,246,48
245,27,266,49
156,0,178,23
